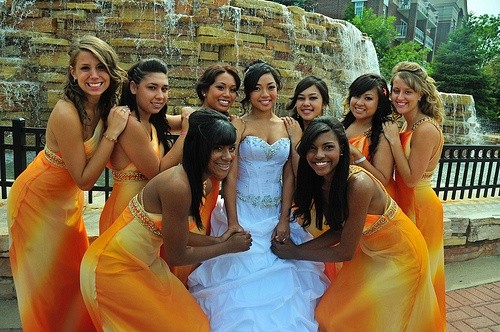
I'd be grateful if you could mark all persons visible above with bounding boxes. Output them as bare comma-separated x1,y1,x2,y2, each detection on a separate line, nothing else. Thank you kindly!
286,76,344,283
382,62,446,332
271,116,443,332
7,35,132,332
160,66,245,291
80,109,253,332
99,58,196,237
341,73,398,203
187,57,332,332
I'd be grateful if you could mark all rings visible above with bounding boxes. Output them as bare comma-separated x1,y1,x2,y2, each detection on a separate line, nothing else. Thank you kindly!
122,109,126,112
282,238,286,242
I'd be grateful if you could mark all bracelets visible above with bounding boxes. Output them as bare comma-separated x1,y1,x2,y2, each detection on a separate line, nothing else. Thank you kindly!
354,156,366,163
179,130,188,135
102,133,118,143
